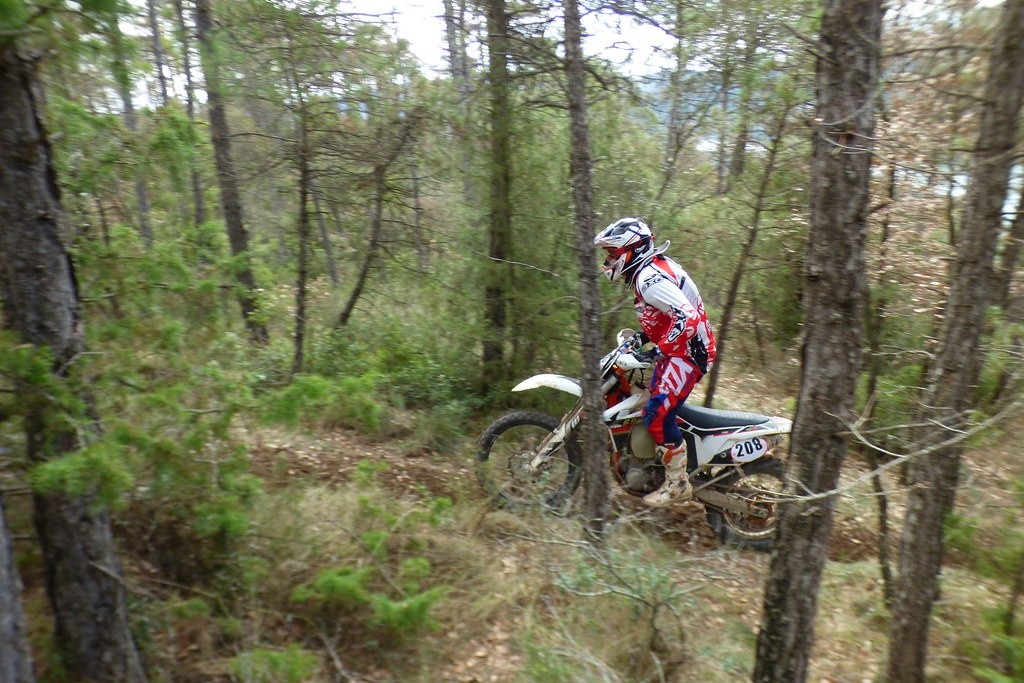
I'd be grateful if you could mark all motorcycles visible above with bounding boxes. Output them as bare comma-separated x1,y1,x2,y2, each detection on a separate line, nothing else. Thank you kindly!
475,331,792,553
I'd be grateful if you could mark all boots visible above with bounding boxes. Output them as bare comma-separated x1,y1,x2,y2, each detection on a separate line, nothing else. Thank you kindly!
642,437,693,507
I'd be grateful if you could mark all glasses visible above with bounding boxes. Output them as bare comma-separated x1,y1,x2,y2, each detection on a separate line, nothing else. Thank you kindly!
602,247,626,258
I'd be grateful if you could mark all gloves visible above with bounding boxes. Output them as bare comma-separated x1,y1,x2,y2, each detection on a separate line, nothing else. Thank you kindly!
620,331,652,354
633,345,666,362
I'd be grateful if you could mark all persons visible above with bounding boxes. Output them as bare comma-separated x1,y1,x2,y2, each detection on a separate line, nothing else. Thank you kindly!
596,211,717,506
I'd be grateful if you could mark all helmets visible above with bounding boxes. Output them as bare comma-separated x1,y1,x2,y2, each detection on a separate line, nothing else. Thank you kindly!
593,215,655,282
